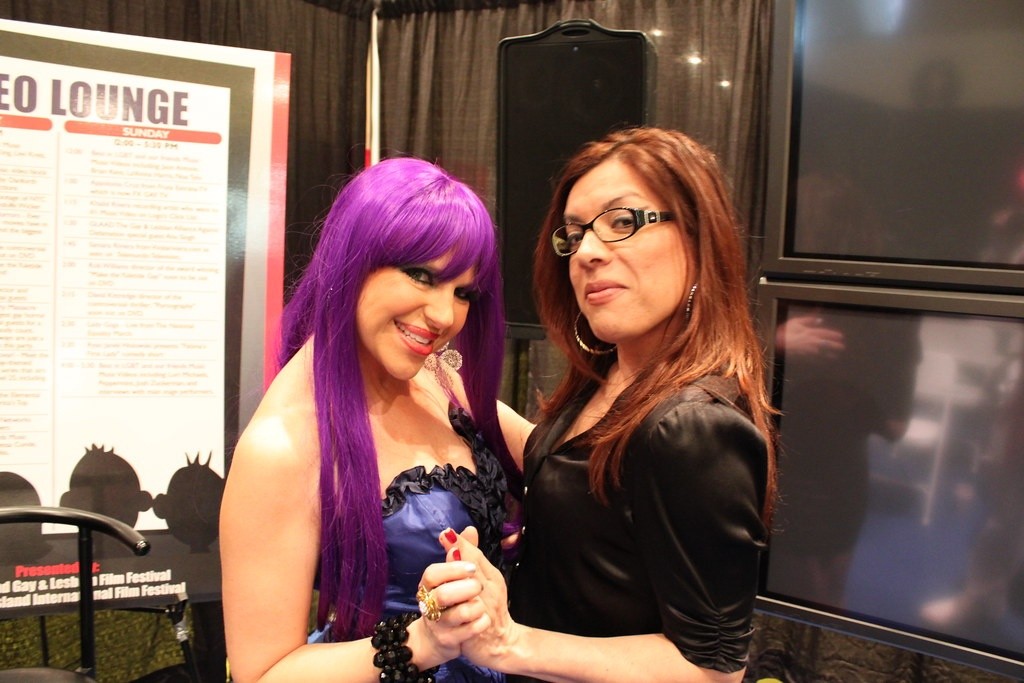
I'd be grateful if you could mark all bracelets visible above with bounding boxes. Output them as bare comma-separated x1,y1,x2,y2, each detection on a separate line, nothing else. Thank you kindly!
370,611,440,683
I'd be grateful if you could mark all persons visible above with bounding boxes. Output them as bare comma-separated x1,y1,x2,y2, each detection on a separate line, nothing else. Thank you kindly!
218,158,522,683
773,162,924,612
919,367,1024,636
439,127,783,683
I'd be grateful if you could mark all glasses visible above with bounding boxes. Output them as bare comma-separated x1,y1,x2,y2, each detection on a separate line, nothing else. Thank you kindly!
552,207,675,257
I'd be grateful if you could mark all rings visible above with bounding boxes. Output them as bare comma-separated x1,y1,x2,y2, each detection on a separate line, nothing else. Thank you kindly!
820,340,832,353
416,586,447,622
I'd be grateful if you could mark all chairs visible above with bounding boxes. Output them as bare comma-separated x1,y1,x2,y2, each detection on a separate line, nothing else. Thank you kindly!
0,505,152,683
